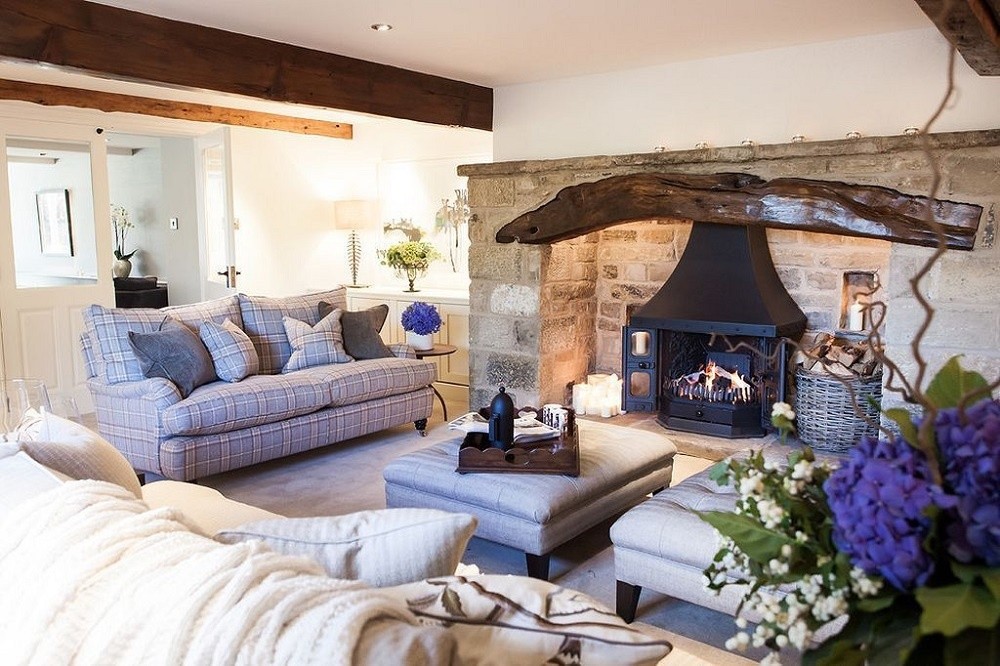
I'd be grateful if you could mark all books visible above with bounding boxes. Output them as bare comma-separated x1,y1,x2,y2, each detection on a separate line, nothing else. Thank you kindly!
448,412,561,444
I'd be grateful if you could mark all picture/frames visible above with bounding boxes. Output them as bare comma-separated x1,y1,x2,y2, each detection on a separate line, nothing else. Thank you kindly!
35,189,74,256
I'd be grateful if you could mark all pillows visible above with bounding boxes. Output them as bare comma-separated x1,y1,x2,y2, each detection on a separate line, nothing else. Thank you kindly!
128,300,397,398
373,574,672,666
20,406,142,500
213,507,479,589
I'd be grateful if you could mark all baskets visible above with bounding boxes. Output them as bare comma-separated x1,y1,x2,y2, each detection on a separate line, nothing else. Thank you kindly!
796,366,884,452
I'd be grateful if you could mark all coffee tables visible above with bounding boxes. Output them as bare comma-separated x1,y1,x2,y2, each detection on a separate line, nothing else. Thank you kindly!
412,344,457,420
384,419,677,584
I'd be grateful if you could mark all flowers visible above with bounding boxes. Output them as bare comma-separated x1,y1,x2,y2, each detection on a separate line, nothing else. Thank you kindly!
401,301,446,336
378,241,441,277
683,354,1000,665
110,202,139,261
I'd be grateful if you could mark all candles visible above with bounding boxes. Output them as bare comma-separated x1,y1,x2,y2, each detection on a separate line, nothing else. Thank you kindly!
571,372,623,417
850,299,864,331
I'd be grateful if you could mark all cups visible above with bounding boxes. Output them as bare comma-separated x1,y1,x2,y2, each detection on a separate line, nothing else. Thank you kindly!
543,403,562,425
550,409,569,434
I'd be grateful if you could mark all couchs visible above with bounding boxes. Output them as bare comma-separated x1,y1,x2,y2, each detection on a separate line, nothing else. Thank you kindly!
610,448,850,643
0,404,760,666
77,286,437,485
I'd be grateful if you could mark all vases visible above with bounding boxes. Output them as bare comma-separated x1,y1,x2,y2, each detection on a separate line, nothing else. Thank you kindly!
402,265,421,293
114,260,131,278
405,331,433,350
